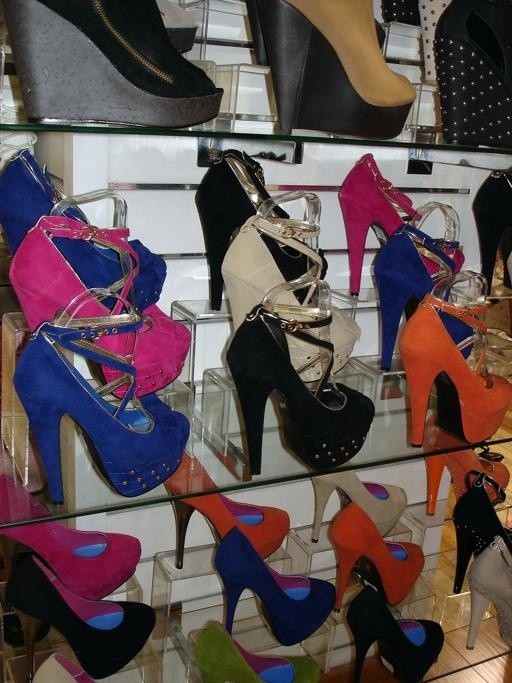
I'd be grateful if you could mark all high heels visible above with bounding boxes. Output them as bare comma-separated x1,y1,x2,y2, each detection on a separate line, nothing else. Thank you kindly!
193,619,320,683
163,451,290,569
1,474,143,601
245,1,417,139
326,503,424,612
344,586,444,682
1,550,157,683
451,485,512,594
308,462,412,544
1,1,225,128
214,525,336,646
465,534,512,649
419,411,512,515
381,0,512,151
194,150,376,477
338,154,512,446
0,149,192,505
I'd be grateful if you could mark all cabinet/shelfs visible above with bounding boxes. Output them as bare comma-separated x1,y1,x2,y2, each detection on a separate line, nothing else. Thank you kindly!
1,120,510,683
